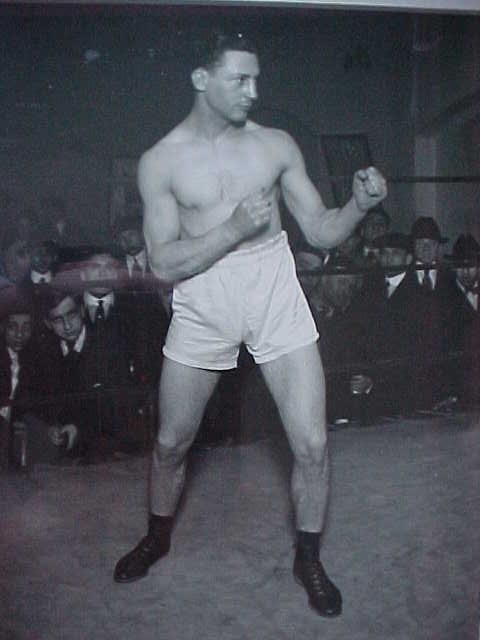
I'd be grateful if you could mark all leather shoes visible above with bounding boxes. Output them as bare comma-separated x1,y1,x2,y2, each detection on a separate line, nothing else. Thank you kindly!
293,552,339,617
114,532,170,583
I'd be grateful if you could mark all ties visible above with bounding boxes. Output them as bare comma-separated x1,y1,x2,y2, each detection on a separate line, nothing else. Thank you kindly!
94,301,105,328
131,256,143,280
423,269,432,293
65,343,77,371
37,278,47,288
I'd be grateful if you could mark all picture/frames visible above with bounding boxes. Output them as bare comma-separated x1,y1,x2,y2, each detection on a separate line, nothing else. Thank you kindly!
321,134,381,208
108,153,144,228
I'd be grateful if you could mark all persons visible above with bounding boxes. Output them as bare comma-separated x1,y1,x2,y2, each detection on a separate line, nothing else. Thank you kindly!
114,36,387,619
0,189,480,472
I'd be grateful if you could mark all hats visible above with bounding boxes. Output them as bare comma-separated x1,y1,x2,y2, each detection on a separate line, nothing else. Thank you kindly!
444,233,479,261
409,218,447,243
368,201,391,224
373,233,407,248
